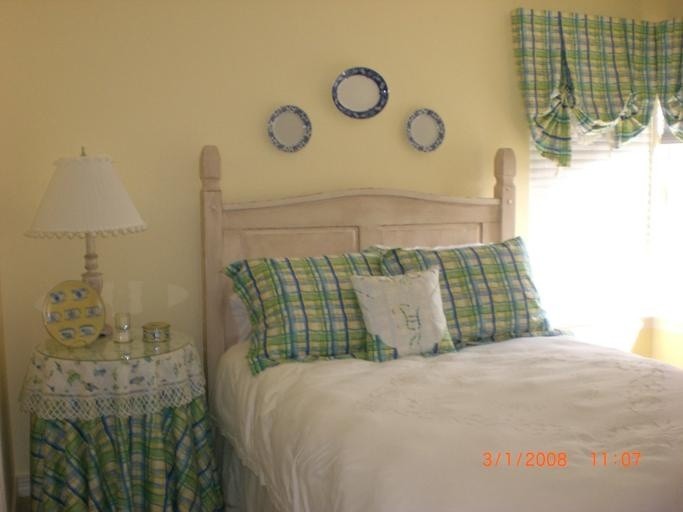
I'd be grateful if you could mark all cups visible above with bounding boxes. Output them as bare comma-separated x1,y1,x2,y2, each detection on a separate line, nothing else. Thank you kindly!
113,313,130,343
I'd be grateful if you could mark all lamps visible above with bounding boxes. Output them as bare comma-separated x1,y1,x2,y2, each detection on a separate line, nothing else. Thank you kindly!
23,146,148,338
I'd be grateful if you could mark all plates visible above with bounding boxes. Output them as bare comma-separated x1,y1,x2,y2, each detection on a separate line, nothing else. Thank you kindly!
268,105,313,153
331,67,389,120
42,279,106,351
408,109,445,153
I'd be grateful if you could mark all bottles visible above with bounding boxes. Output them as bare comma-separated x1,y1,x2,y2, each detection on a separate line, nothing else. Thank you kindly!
140,322,170,344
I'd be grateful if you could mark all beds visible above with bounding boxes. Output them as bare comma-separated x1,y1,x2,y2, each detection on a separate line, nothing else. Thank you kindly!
200,145,683,512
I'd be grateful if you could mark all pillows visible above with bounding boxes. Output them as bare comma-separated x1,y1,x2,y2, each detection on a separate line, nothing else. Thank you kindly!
218,251,380,376
379,236,550,348
351,265,456,362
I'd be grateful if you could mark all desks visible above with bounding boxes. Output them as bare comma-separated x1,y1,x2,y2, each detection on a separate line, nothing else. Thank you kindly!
18,326,220,512
